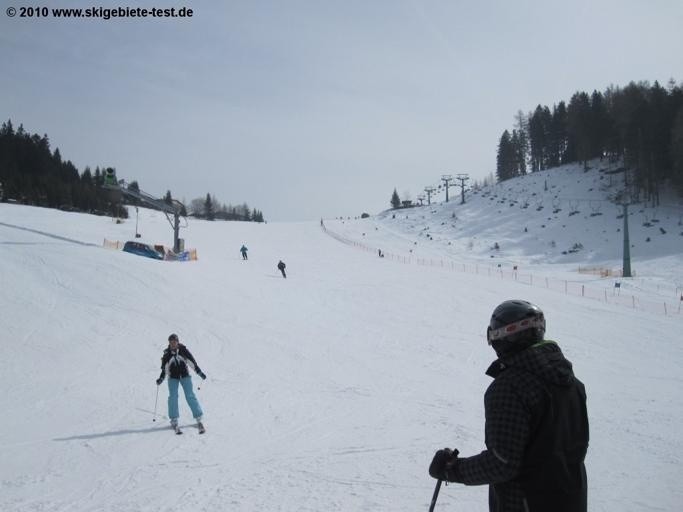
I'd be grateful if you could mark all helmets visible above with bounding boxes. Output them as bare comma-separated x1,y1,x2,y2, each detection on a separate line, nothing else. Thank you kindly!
488,300,547,358
168,334,178,341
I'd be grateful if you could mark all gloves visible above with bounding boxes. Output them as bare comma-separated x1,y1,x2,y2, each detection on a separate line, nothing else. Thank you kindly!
157,379,163,385
201,373,206,379
429,447,458,485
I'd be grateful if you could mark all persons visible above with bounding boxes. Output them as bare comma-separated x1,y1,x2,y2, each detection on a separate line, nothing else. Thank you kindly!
428,299,589,512
240,244,249,260
378,248,382,256
278,260,286,278
155,334,206,434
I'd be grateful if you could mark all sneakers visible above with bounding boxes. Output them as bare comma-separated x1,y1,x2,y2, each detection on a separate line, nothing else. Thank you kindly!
198,422,205,434
174,426,182,434
